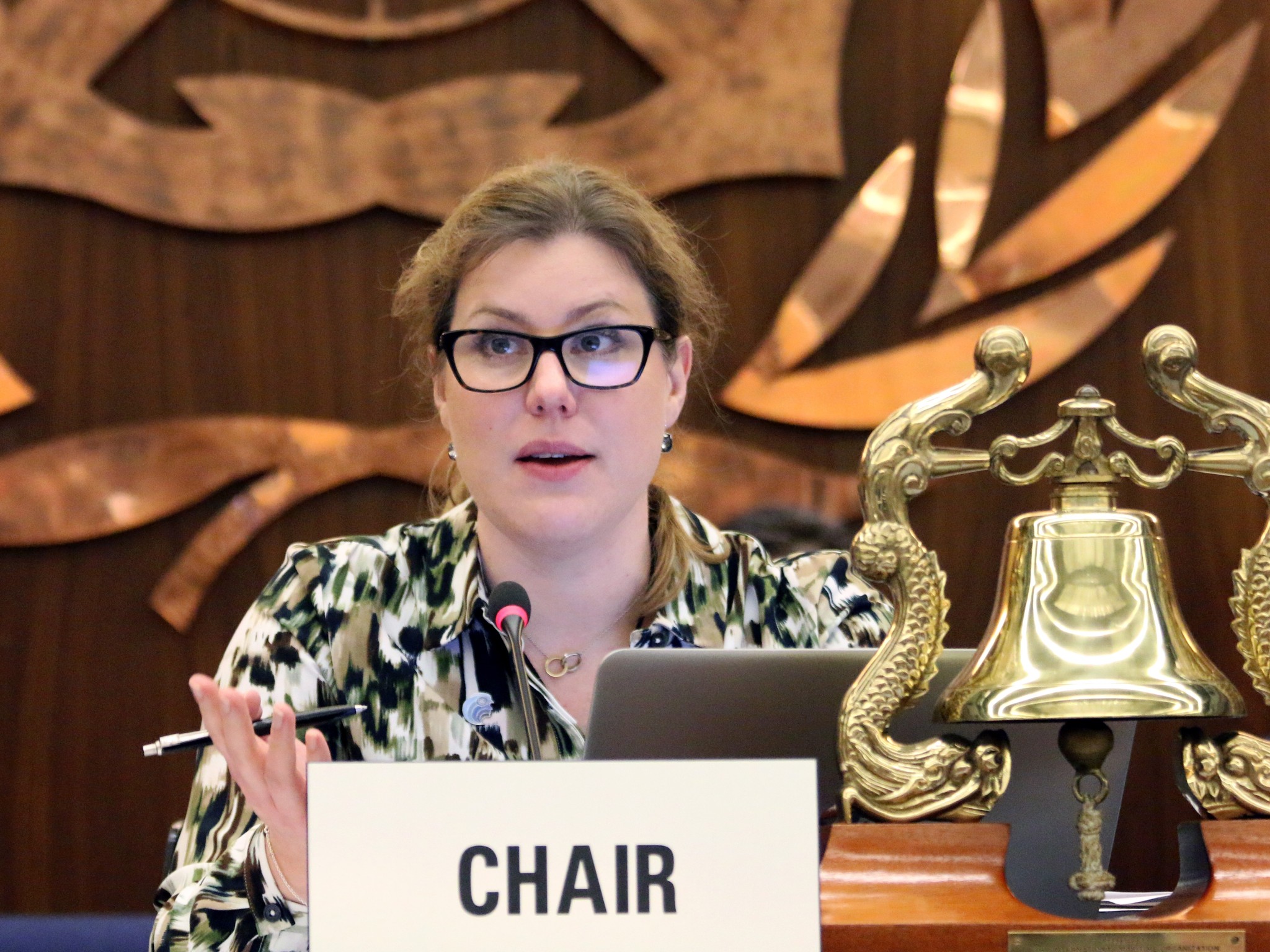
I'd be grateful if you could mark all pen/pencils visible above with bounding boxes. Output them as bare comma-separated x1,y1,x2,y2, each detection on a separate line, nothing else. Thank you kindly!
141,703,368,757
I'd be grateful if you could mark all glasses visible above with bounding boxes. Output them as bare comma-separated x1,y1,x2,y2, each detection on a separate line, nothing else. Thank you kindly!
436,325,673,393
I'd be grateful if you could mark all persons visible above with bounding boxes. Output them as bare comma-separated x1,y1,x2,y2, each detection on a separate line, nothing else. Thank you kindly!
148,157,894,952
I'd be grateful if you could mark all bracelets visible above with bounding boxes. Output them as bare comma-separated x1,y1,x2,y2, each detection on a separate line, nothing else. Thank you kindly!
264,825,307,907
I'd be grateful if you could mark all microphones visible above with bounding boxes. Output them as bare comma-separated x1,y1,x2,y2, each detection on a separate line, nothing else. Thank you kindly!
491,581,541,759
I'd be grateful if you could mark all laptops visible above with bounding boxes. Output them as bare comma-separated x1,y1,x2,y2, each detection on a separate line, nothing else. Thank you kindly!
584,650,1000,823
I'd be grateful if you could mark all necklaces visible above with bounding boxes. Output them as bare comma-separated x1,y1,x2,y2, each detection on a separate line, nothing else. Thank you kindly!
522,583,649,678
